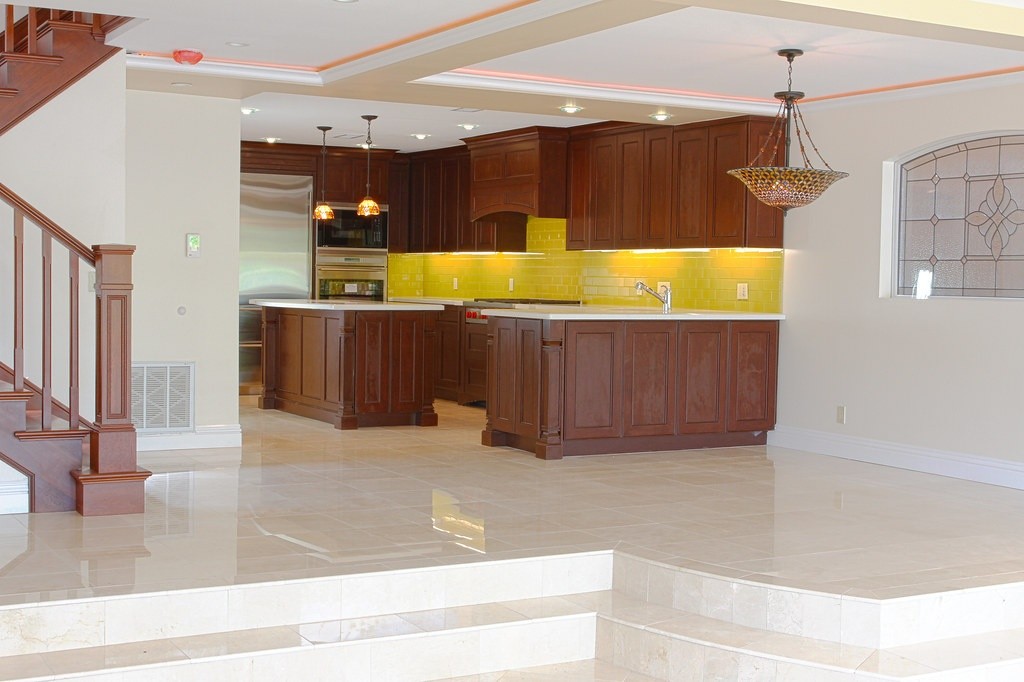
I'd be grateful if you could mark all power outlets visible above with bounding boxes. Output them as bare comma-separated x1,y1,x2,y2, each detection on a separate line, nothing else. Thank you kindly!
736,282,747,299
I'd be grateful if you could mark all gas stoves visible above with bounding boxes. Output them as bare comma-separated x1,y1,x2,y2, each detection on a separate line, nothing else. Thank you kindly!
464,297,583,325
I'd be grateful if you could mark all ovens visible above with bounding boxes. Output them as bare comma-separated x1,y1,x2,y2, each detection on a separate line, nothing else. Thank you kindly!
312,265,388,303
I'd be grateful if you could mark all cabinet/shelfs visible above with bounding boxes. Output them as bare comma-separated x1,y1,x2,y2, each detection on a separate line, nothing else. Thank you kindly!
241,114,789,254
260,306,781,462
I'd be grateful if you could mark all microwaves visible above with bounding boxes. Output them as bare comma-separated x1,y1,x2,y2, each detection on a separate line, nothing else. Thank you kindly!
315,201,389,254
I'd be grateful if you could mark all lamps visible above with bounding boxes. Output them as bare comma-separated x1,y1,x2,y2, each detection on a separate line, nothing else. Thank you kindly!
312,126,334,219
172,50,202,65
356,115,379,216
726,49,850,219
